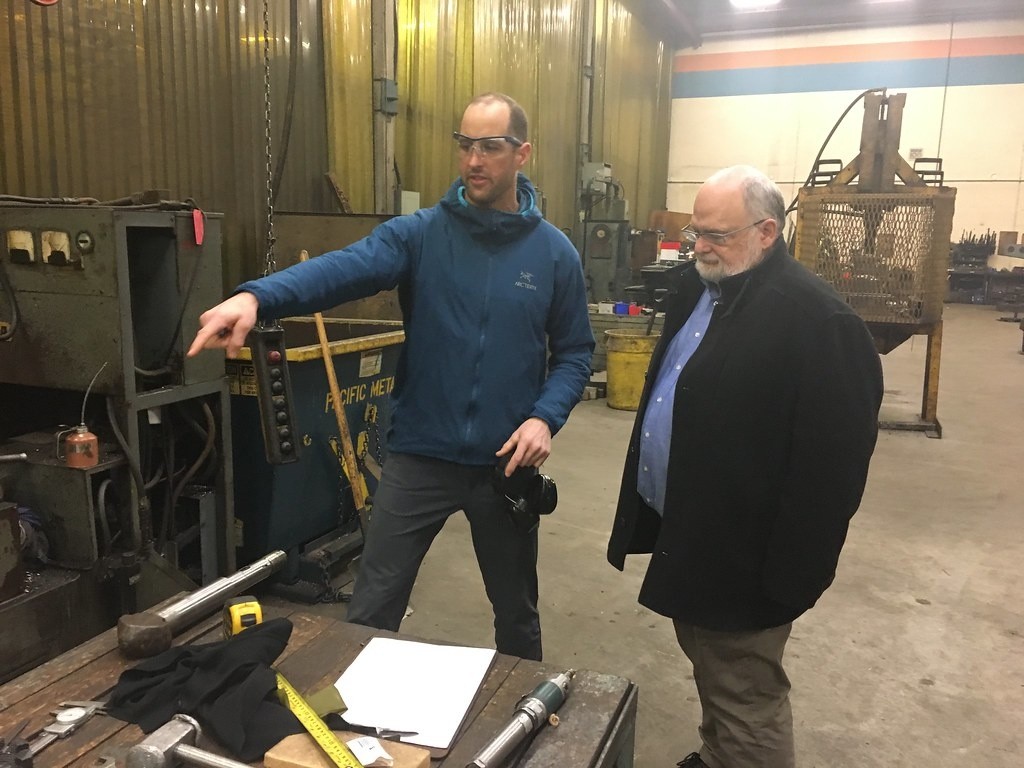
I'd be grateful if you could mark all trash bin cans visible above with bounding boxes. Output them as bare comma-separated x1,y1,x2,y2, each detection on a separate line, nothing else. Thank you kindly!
603,326,662,414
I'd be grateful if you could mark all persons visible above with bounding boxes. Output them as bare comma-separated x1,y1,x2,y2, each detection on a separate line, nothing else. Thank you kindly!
606,164,885,768
187,93,596,662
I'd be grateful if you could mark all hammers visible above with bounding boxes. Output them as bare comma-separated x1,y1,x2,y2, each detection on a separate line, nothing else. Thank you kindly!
124,711,254,768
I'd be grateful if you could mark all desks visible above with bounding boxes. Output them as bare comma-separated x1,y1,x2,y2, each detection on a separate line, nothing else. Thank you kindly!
1,593,640,768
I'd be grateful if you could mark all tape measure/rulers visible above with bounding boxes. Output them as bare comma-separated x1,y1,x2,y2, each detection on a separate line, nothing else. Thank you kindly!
221,594,367,768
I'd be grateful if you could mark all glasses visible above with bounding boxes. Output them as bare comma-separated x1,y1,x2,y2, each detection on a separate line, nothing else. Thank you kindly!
680,216,772,245
451,132,523,161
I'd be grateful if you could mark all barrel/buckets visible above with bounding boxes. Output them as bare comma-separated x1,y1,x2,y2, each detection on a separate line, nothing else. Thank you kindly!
603,328,664,411
